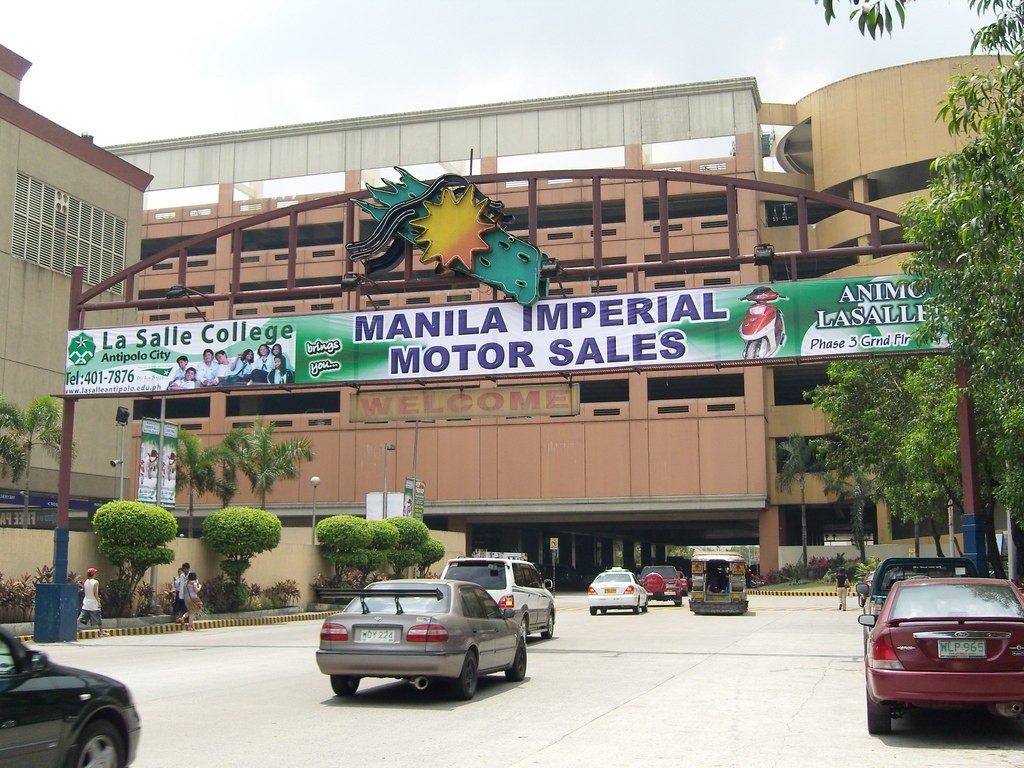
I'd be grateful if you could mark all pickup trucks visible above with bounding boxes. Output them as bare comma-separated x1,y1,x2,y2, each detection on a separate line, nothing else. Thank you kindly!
855,557,988,661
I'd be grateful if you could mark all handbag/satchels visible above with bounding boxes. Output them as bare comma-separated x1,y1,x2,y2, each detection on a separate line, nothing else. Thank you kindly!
844,574,850,588
191,598,203,612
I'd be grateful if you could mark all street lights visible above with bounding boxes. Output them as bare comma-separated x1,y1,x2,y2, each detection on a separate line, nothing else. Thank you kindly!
382,443,398,518
311,476,319,544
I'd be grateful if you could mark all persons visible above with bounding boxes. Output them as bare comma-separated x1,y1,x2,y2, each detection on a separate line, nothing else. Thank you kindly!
173,562,202,631
835,567,850,611
76,568,109,636
168,343,292,389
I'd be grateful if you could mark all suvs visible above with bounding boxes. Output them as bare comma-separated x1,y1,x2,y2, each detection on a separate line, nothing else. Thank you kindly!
439,555,556,643
638,566,684,607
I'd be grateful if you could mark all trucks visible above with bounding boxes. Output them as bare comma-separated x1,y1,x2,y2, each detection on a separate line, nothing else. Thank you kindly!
688,550,749,614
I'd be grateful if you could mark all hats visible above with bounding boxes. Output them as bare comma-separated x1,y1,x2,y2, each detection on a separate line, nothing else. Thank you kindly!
87,568,98,577
186,573,198,580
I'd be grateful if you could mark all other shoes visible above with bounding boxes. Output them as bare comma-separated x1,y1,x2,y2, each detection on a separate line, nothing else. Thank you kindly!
839,603,842,610
179,618,185,625
99,631,110,637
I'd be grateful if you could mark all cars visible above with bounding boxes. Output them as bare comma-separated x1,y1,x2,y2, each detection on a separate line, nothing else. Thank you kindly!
0,627,143,768
676,570,689,597
315,578,527,702
587,566,649,615
859,571,875,607
857,576,1024,735
